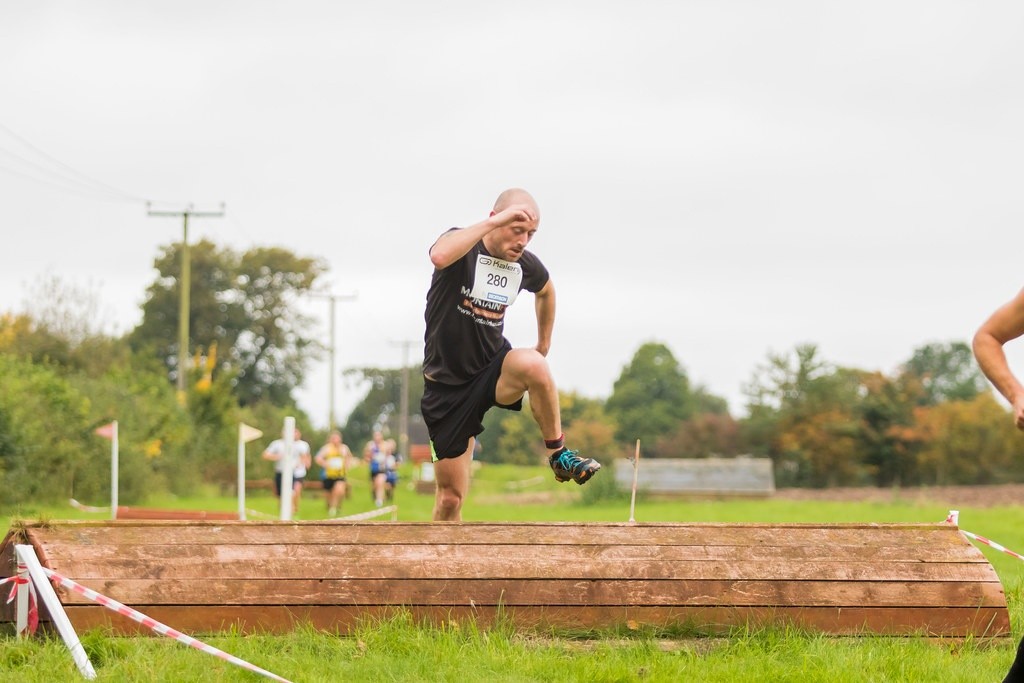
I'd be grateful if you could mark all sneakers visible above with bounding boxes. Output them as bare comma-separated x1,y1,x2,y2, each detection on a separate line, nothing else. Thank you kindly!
548,447,601,485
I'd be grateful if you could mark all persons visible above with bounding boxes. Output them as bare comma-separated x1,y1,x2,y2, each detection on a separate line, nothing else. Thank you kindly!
420,188,603,522
362,431,392,506
314,429,353,515
972,287,1024,683
382,438,401,506
262,427,313,522
